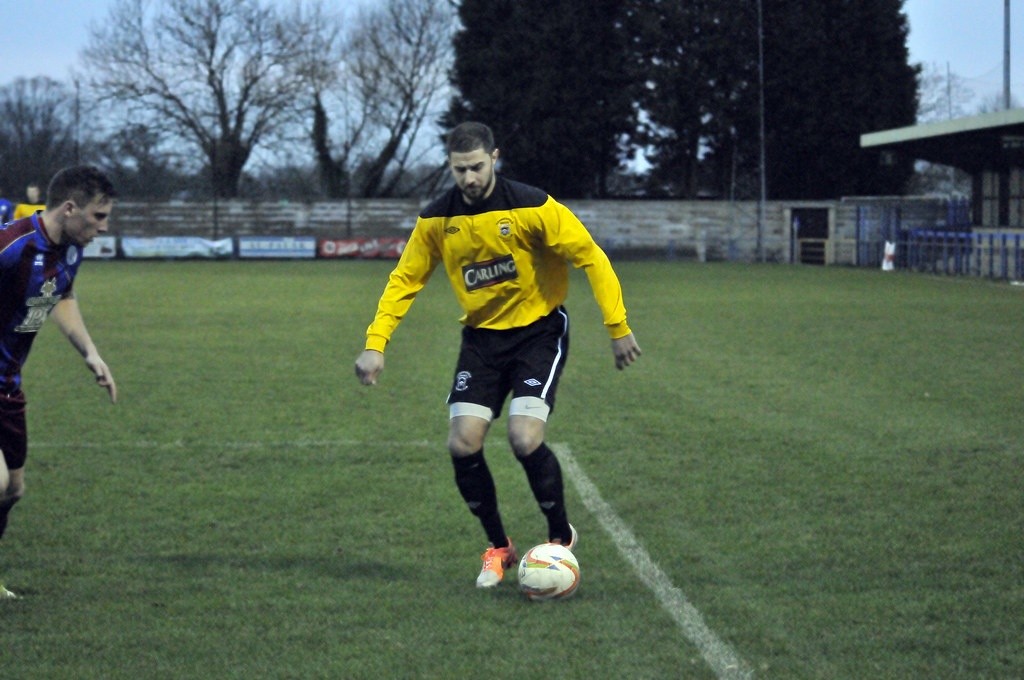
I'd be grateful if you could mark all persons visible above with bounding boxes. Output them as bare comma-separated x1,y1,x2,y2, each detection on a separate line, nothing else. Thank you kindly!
14,184,47,220
0,199,13,226
354,123,643,588
0,165,119,599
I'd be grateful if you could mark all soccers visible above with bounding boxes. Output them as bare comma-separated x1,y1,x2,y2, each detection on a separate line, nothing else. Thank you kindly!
516,541,580,602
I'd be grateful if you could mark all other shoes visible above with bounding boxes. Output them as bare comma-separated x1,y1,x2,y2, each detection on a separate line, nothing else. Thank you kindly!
0,584,16,600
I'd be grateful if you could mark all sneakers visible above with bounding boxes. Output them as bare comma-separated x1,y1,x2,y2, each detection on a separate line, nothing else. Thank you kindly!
548,522,577,551
476,536,518,587
